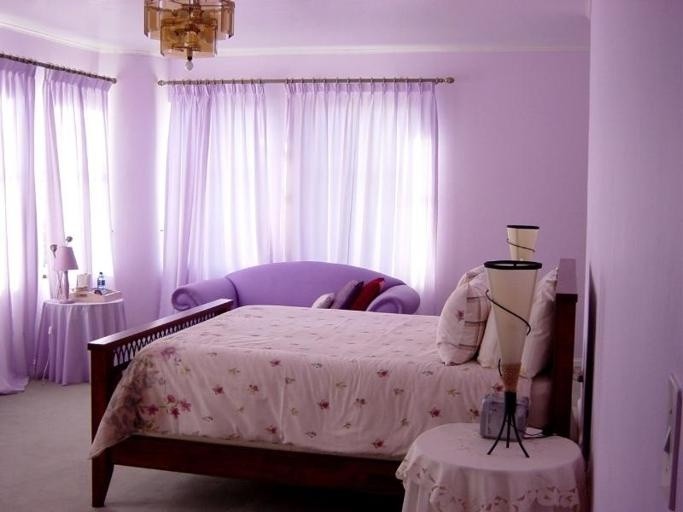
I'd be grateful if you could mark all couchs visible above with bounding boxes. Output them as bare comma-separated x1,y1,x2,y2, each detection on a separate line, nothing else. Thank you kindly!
171,260,420,315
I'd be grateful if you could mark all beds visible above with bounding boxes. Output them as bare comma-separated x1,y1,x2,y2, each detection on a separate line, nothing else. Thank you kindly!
86,257,579,509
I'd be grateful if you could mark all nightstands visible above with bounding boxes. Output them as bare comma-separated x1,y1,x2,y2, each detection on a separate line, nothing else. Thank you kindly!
402,423,586,511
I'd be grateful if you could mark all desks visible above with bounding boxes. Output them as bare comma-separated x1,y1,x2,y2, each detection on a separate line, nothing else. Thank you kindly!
40,297,128,387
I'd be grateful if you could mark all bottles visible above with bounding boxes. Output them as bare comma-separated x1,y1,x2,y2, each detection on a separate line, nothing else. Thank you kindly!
96,272,107,296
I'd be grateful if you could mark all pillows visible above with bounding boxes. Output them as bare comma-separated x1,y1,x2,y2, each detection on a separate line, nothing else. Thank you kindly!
309,274,385,312
435,261,558,371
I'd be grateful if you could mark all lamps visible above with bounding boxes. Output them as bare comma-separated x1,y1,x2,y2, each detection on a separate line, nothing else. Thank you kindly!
50,246,79,304
505,224,539,266
485,257,544,463
144,1,235,74
